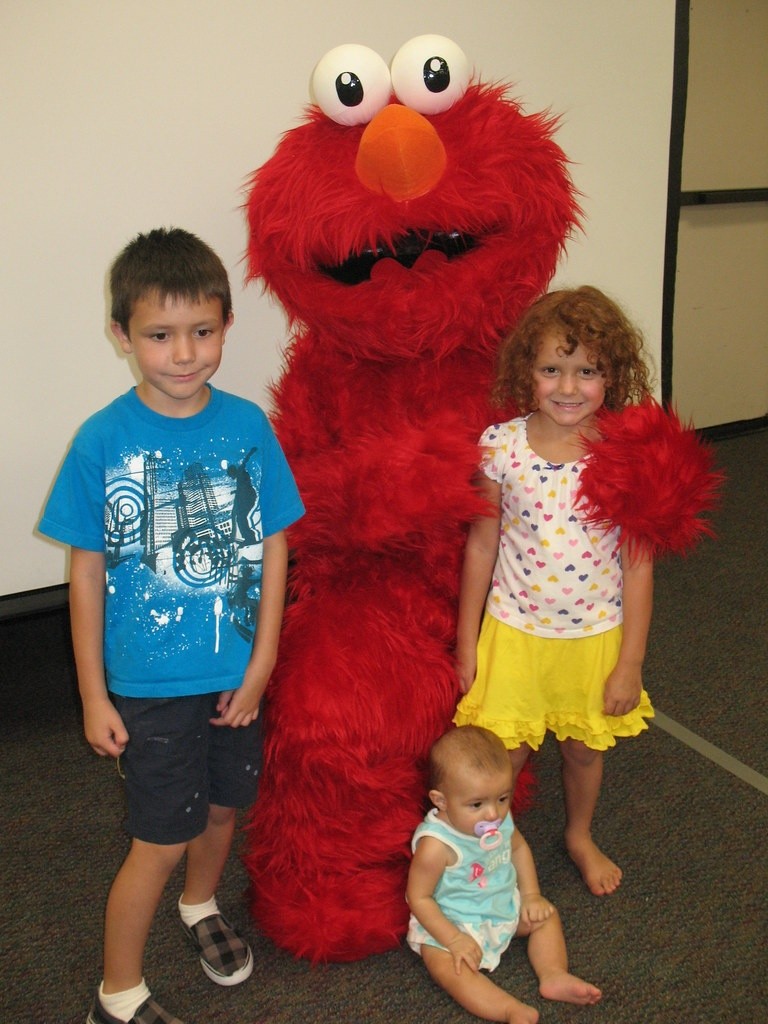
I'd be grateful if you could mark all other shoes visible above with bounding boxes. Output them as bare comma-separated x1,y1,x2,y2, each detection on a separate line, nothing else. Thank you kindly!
85,984,184,1024
179,907,255,987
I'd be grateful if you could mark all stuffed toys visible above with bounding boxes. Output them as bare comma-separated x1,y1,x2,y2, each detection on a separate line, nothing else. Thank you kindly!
240,32,723,966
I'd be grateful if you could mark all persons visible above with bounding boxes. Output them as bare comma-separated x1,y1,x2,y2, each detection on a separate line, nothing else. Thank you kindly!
36,227,308,1024
405,725,602,1024
454,285,657,897
